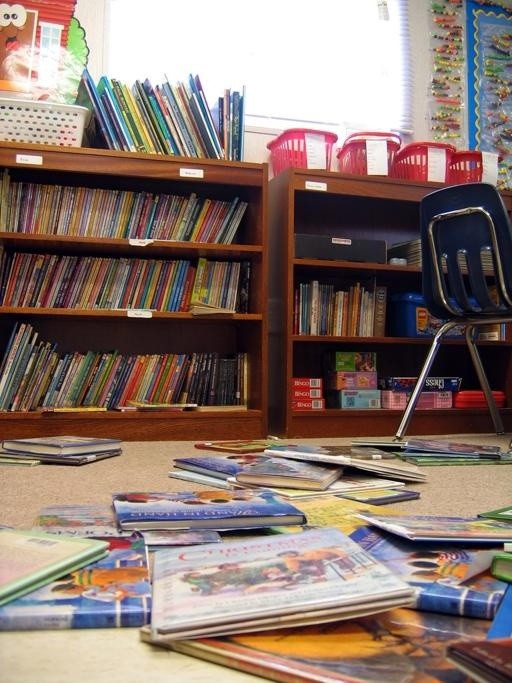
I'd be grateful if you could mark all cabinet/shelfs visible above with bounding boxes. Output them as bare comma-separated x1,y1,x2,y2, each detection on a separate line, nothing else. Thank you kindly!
0,141,267,442
268,167,512,438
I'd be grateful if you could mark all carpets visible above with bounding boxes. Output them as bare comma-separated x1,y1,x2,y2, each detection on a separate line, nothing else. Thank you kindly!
0,434,512,683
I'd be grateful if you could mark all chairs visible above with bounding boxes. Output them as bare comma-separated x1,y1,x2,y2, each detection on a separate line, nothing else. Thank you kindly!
396,183,512,439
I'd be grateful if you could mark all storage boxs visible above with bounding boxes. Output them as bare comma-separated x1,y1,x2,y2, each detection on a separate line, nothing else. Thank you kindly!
398,290,481,338
267,128,338,176
394,142,457,184
451,151,503,184
0,97,91,147
336,132,402,176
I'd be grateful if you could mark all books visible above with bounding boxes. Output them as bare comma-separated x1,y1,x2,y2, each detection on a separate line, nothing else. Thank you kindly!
1,168,251,246
79,70,248,162
293,225,493,269
295,279,389,339
139,505,510,682
0,490,420,641
0,434,124,468
169,435,511,491
0,318,250,412
1,249,253,315
